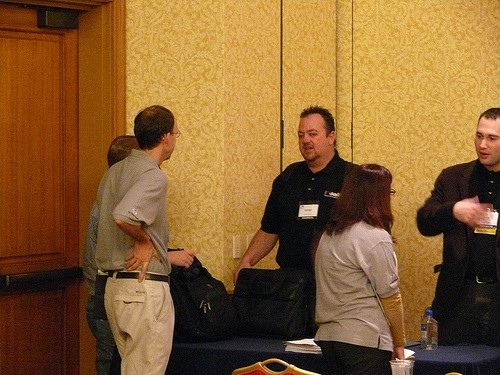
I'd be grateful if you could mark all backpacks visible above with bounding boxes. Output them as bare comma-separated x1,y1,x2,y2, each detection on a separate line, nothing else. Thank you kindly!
166,248,237,341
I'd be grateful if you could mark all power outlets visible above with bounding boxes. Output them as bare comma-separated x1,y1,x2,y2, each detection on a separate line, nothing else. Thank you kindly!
233,235,241,258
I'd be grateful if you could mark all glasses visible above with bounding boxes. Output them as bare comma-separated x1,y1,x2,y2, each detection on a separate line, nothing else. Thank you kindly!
160,130,181,138
390,189,396,196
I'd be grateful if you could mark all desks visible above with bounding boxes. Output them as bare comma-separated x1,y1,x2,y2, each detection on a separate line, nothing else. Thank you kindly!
166,335,500,375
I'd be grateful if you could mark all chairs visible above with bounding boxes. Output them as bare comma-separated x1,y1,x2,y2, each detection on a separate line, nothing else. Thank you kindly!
231,268,314,340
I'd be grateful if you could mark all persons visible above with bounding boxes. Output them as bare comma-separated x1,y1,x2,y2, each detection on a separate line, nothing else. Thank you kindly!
313,164,405,375
84,135,195,375
416,108,500,345
95,105,182,375
232,107,362,287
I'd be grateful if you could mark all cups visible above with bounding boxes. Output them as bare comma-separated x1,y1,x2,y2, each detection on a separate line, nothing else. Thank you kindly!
389,359,415,375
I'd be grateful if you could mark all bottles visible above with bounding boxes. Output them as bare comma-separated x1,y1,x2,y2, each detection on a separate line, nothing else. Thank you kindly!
420,310,437,350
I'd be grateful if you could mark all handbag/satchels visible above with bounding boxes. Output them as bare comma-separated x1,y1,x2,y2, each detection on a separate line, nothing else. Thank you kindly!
231,268,312,340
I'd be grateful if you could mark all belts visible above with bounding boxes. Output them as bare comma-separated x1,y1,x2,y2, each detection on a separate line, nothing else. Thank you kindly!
469,274,497,284
108,271,169,283
